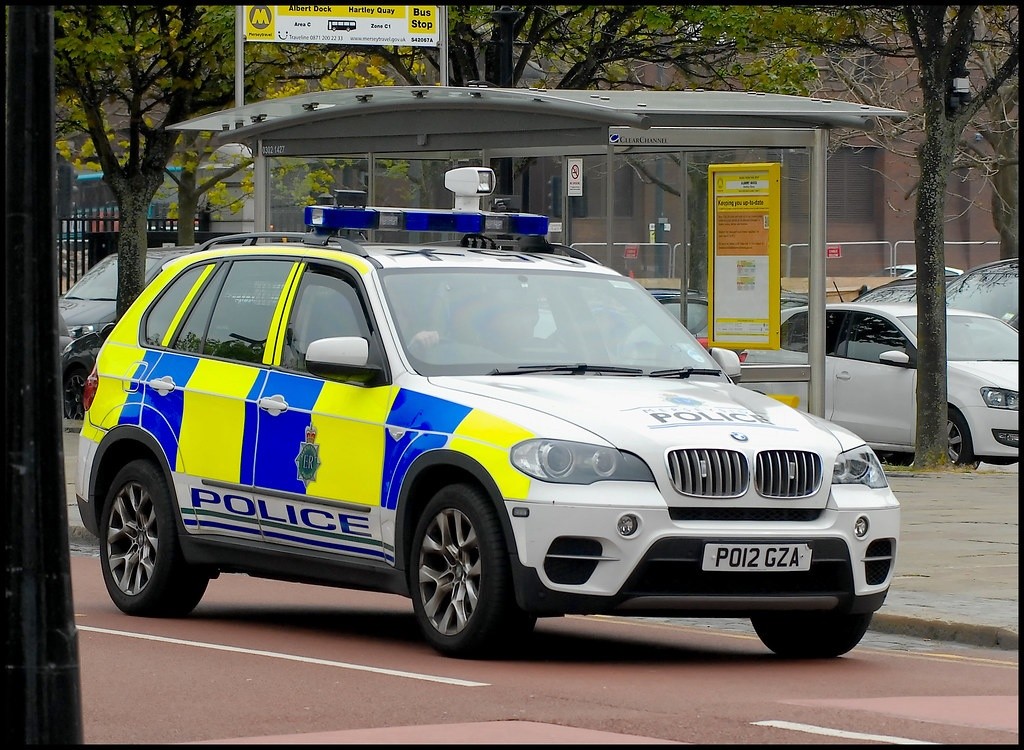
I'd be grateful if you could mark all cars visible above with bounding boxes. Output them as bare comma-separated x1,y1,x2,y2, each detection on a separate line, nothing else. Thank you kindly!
643,258,1019,471
60,246,234,433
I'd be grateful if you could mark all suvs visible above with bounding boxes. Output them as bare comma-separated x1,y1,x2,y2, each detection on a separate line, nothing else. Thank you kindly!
73,164,903,663
